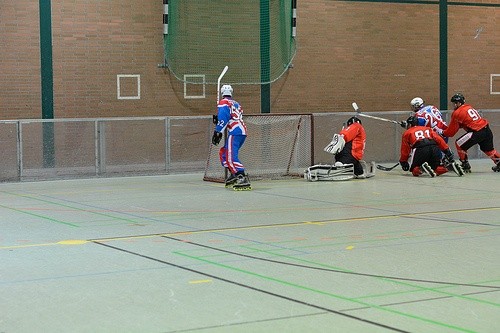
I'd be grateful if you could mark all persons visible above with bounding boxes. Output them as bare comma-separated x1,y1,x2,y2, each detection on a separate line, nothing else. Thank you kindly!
400,116,465,178
438,93,500,173
325,117,365,179
212,85,252,191
410,97,448,144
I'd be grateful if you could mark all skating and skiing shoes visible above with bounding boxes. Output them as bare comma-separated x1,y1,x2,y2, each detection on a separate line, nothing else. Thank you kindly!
225,172,238,189
233,174,252,191
492,160,500,172
447,161,465,176
422,161,436,178
459,154,471,173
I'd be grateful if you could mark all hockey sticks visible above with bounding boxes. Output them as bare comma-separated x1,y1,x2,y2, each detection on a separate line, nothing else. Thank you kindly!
351,102,403,125
217,65,229,106
376,154,413,172
342,122,346,131
431,109,447,127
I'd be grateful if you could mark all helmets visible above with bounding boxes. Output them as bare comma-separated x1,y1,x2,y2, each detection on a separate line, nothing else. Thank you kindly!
347,117,362,126
221,84,234,97
450,94,466,105
410,97,424,110
406,116,419,126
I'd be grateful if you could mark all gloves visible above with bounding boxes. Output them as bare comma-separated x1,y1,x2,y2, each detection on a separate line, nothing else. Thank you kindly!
436,128,446,139
212,131,222,145
399,120,408,129
443,148,455,163
213,114,218,125
399,160,409,171
